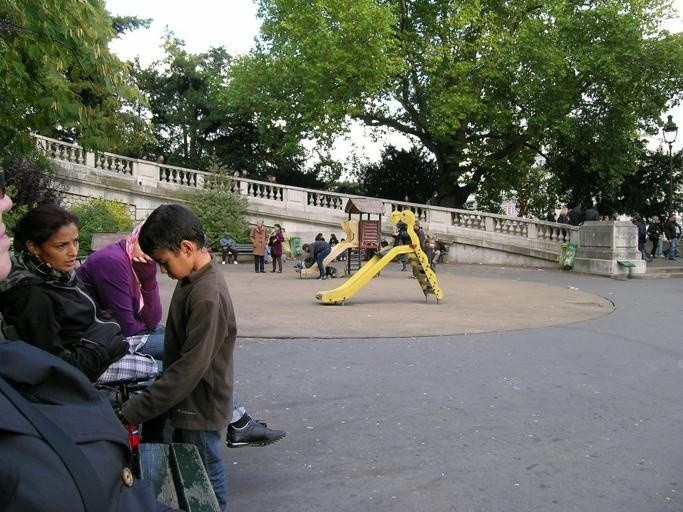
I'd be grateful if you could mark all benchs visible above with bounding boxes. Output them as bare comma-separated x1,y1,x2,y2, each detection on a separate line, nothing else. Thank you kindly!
228,243,254,265
131,443,222,512
438,249,445,262
76,255,88,264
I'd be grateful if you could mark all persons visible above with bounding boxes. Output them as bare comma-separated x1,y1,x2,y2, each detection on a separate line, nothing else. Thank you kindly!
545,207,682,263
249,216,451,280
0,168,189,511
2,204,287,447
154,154,164,165
75,218,169,355
114,201,235,512
429,190,440,206
232,167,279,198
219,229,239,265
139,152,148,161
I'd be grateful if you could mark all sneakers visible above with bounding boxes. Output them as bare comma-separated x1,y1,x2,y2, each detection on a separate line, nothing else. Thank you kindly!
272,269,282,273
662,250,680,261
316,276,326,280
233,260,238,264
225,419,287,448
222,261,226,264
648,252,655,263
256,270,266,273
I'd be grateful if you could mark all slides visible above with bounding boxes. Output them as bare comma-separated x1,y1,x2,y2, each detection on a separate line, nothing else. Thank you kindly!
314,245,413,303
300,239,360,279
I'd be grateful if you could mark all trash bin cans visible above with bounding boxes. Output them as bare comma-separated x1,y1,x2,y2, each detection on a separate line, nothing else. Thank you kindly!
288,236,301,257
560,243,578,270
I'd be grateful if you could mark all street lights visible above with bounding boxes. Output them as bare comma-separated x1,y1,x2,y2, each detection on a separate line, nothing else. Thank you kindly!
662,114,679,216
